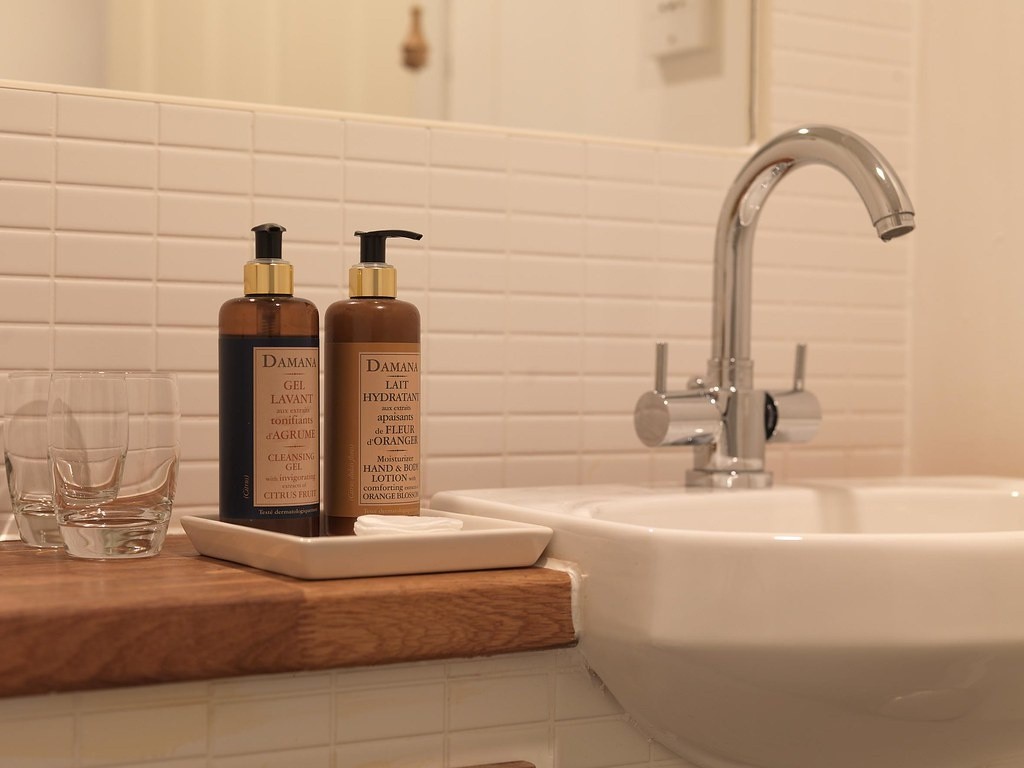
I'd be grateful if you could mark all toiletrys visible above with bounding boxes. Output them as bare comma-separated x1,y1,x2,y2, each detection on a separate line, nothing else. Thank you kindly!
219,223,323,539
324,229,423,536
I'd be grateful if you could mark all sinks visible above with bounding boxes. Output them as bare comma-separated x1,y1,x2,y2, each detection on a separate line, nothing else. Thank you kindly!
430,470,1024,765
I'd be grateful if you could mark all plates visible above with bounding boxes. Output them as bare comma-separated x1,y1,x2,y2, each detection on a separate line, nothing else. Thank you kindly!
183,508,555,579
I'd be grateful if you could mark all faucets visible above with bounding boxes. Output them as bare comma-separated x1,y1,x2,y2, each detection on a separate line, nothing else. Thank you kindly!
704,123,914,389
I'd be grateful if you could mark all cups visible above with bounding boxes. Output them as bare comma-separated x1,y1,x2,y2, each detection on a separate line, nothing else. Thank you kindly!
0,371,129,549
48,371,180,559
